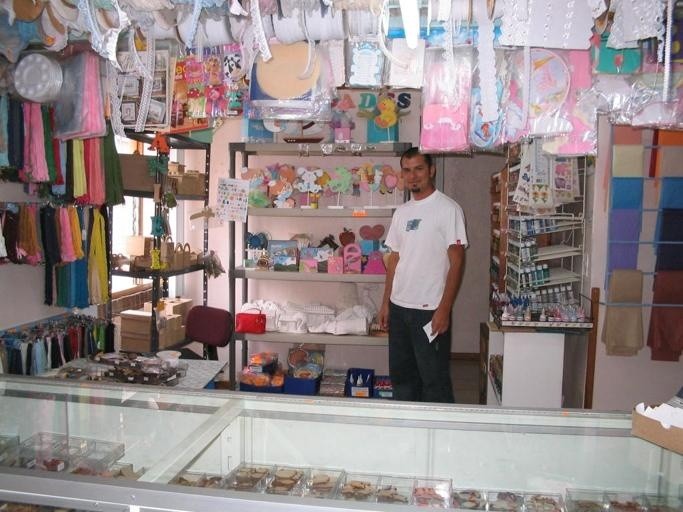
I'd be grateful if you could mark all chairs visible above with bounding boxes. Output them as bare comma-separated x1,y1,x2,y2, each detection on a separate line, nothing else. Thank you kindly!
175,306,235,362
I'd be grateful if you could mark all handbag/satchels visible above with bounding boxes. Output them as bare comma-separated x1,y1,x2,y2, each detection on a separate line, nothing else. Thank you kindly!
236,309,266,333
160,236,191,270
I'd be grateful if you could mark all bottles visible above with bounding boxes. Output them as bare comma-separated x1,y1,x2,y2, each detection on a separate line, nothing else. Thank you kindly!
505,216,576,305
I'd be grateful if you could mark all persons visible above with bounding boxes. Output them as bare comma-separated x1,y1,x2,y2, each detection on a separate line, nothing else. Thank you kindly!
374,145,470,405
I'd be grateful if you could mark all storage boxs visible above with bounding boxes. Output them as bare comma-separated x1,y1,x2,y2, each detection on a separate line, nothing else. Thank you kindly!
119,298,194,354
116,154,207,197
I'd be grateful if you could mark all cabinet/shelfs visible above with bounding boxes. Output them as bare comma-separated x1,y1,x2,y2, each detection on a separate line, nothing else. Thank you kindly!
228,142,412,390
0,375,683,510
106,125,209,353
480,161,592,410
